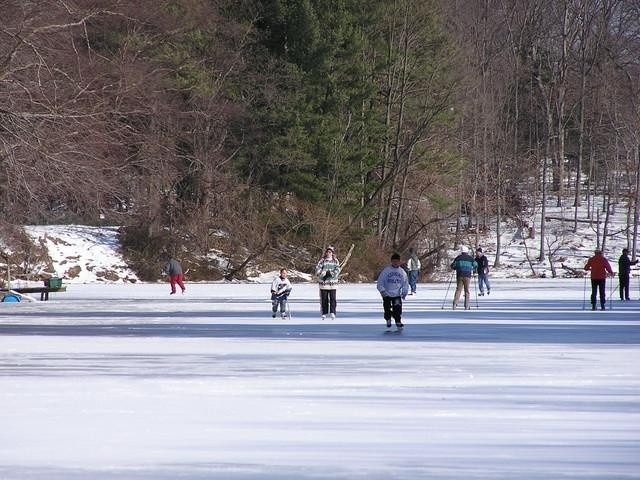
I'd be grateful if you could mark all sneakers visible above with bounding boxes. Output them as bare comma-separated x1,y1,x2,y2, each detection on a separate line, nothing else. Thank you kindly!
280,311,286,317
592,304,605,310
272,312,277,318
452,304,458,309
464,305,469,309
386,319,392,328
330,312,336,318
321,312,328,319
396,321,405,328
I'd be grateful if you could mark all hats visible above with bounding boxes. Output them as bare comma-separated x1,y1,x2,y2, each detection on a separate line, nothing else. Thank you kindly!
460,245,469,253
476,248,483,252
327,246,335,252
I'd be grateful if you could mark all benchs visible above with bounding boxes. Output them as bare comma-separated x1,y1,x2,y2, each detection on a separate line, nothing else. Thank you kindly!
1,284,67,300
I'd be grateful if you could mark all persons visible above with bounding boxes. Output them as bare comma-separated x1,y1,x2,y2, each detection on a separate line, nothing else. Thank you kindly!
271,268,292,317
619,248,638,300
473,248,490,295
377,254,408,327
316,246,339,320
164,256,185,295
584,249,613,310
406,247,421,294
450,246,477,310
